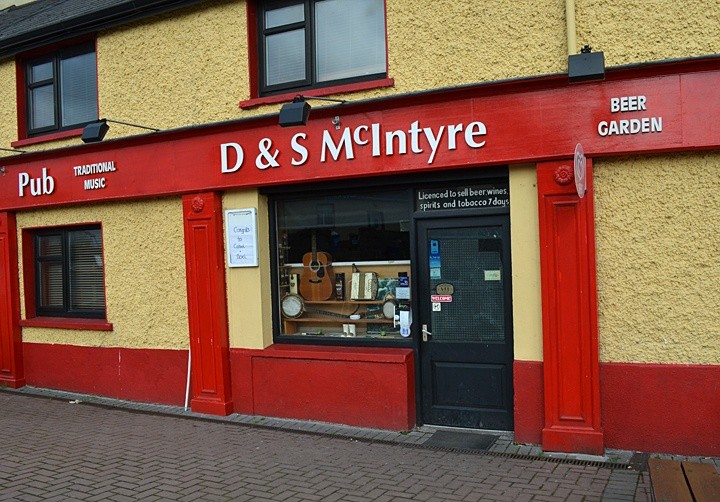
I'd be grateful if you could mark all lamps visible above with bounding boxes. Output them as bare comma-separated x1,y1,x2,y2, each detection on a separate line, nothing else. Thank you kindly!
279,94,350,128
568,45,606,84
81,118,159,143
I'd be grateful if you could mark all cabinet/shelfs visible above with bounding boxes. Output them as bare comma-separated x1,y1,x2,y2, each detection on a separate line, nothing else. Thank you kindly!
278,246,290,300
283,299,395,324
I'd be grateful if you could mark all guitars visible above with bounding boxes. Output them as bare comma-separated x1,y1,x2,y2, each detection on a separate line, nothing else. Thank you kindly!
353,299,399,319
300,230,335,301
280,293,351,320
